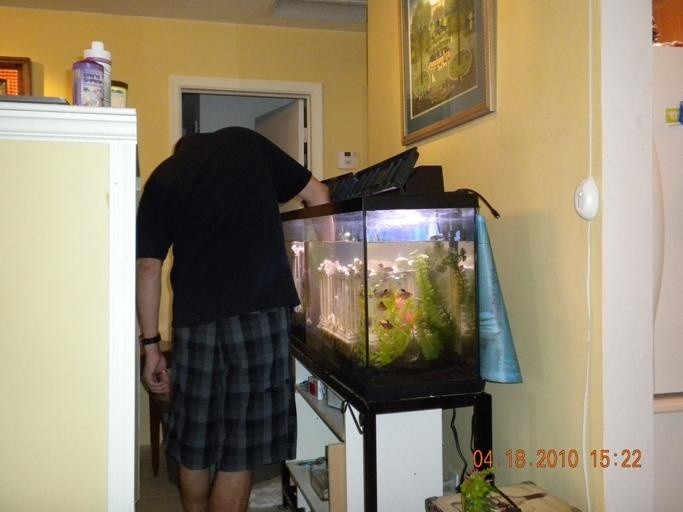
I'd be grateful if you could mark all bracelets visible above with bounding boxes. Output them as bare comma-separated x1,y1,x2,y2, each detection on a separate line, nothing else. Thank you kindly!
142,332,162,345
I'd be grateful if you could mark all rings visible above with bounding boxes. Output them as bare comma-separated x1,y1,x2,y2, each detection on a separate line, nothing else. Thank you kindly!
157,369,167,376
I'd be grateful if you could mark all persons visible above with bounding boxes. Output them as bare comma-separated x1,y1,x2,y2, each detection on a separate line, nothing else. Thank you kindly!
136,124,336,512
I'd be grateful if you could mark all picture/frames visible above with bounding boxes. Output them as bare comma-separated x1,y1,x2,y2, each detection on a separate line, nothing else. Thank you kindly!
399,0,496,146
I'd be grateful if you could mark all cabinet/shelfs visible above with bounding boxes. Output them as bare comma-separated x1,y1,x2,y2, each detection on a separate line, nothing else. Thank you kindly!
281,338,492,512
